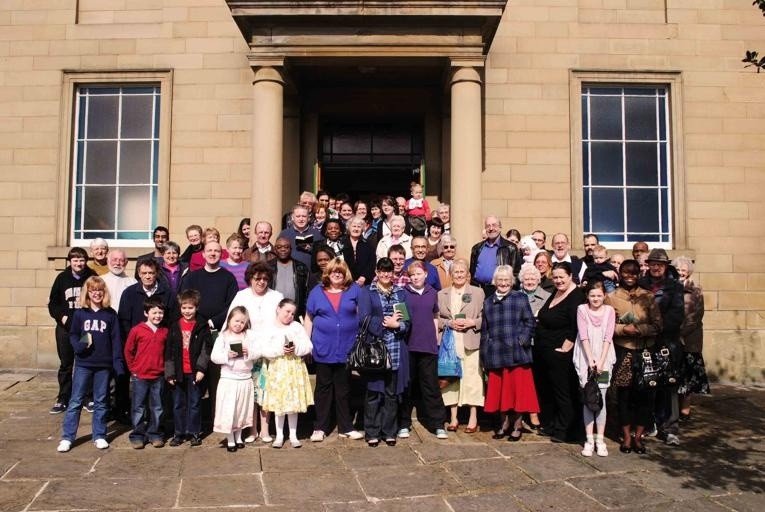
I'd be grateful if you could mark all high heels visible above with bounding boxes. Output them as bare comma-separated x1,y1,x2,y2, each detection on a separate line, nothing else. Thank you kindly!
492,427,508,439
508,428,523,441
531,424,540,429
620,441,631,453
465,423,477,433
679,408,691,421
386,437,396,446
447,422,459,430
368,439,378,446
634,447,645,454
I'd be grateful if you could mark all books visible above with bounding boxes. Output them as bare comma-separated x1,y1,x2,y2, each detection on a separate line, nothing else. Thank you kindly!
285,335,294,348
454,313,467,320
392,302,411,322
294,233,314,246
619,311,638,326
596,370,610,384
229,340,244,358
79,332,93,349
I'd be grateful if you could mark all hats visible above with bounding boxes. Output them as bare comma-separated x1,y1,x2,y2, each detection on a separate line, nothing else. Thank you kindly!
645,248,672,263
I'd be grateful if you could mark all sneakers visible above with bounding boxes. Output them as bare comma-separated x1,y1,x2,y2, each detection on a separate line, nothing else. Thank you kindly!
132,439,144,449
291,440,302,447
595,440,608,457
245,432,258,442
259,431,272,442
581,441,594,456
152,439,164,447
338,430,363,440
83,402,94,413
310,430,326,441
648,423,658,436
436,429,448,438
57,439,71,452
272,442,284,448
169,435,183,445
94,438,108,448
49,400,67,414
191,436,202,445
398,428,409,438
666,434,680,446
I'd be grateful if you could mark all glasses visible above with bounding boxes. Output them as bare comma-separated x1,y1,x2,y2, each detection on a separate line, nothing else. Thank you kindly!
253,276,268,281
444,245,455,249
88,289,103,293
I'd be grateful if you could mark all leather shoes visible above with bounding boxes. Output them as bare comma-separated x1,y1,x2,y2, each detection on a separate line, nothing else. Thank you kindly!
235,440,245,448
538,428,547,436
227,443,237,452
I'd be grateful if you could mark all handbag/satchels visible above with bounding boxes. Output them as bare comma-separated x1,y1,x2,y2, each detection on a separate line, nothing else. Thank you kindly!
346,315,392,372
584,366,603,411
638,323,681,388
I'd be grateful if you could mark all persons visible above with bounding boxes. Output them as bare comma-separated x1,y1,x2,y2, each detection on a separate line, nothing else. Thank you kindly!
336,194,349,211
531,230,554,253
220,231,252,291
520,235,539,264
210,306,262,452
238,217,251,251
579,233,599,263
356,257,413,446
315,191,330,207
375,215,413,263
436,202,451,234
505,228,521,246
429,233,458,289
404,182,432,223
134,226,170,281
158,240,189,301
370,244,411,288
178,241,239,321
404,235,442,292
604,259,666,454
57,275,124,452
47,246,99,414
164,289,214,447
242,220,277,262
398,260,449,439
117,257,176,346
518,262,552,428
86,237,111,275
355,199,377,244
534,250,553,283
550,232,579,278
219,260,285,443
97,248,136,315
536,261,586,444
638,248,686,446
396,195,407,217
438,260,485,433
592,245,619,292
203,227,221,243
469,215,518,298
479,265,537,442
425,217,444,264
303,256,364,443
337,201,353,237
670,255,712,421
276,204,325,269
281,190,318,229
341,216,375,286
328,197,336,210
306,244,338,298
311,203,330,230
123,297,169,449
377,194,400,243
631,241,649,260
353,200,371,230
581,253,624,292
181,224,205,263
571,280,617,457
262,298,315,449
313,218,346,274
265,234,312,322
